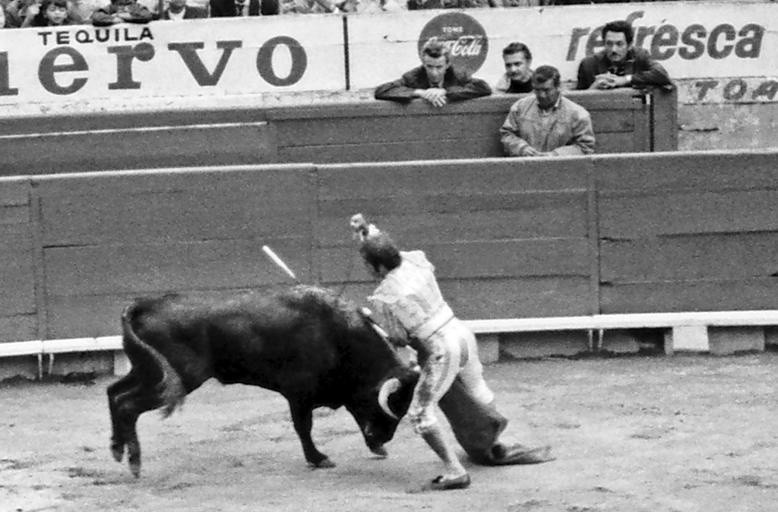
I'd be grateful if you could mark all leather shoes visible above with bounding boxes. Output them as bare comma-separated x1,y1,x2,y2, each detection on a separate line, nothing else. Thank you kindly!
431,475,470,489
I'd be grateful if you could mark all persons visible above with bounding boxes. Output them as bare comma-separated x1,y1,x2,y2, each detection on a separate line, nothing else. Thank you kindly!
575,22,670,89
499,65,596,157
349,212,556,490
0,0,597,27
491,42,535,93
373,37,491,108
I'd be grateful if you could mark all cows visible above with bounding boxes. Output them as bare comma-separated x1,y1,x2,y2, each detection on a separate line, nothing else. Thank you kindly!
106,281,424,480
